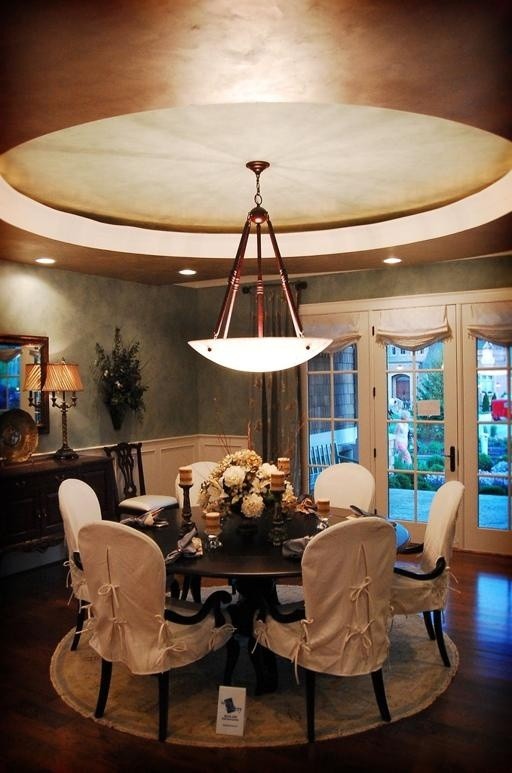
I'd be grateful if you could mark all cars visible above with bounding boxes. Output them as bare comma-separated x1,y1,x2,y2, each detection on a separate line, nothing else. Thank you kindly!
488,398,512,421
0,383,21,409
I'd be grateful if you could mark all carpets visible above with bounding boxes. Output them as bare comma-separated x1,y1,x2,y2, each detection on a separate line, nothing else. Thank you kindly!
48,585,457,748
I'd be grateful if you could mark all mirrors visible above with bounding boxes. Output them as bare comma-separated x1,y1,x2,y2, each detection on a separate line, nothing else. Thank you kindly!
0,334,49,434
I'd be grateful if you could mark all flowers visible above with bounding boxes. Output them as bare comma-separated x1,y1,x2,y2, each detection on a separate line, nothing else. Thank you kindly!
88,325,149,430
199,449,297,522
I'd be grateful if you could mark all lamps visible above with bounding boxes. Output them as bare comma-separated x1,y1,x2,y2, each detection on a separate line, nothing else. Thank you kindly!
20,362,40,411
40,356,83,459
186,159,332,374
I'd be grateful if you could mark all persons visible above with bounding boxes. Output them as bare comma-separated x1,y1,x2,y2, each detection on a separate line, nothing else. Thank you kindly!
395,410,411,463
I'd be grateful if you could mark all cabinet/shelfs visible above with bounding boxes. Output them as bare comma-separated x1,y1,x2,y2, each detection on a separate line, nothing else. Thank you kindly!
0,453,121,555
